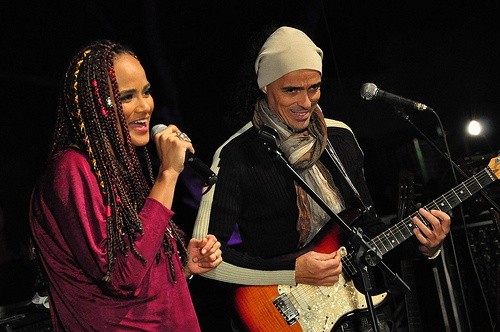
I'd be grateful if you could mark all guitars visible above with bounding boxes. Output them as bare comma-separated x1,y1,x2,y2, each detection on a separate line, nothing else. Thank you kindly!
216,153,500,332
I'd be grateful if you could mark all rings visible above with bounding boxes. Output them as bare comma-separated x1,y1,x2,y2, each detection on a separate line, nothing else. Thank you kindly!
176,132,191,142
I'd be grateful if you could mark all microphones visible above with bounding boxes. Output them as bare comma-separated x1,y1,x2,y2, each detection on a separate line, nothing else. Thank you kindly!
361,83,433,112
151,124,217,186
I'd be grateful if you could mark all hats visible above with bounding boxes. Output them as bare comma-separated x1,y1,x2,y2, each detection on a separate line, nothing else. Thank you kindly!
255,26,324,89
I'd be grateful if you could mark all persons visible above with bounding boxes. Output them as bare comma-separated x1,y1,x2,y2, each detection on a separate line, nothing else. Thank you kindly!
192,25,451,332
29,39,223,332
